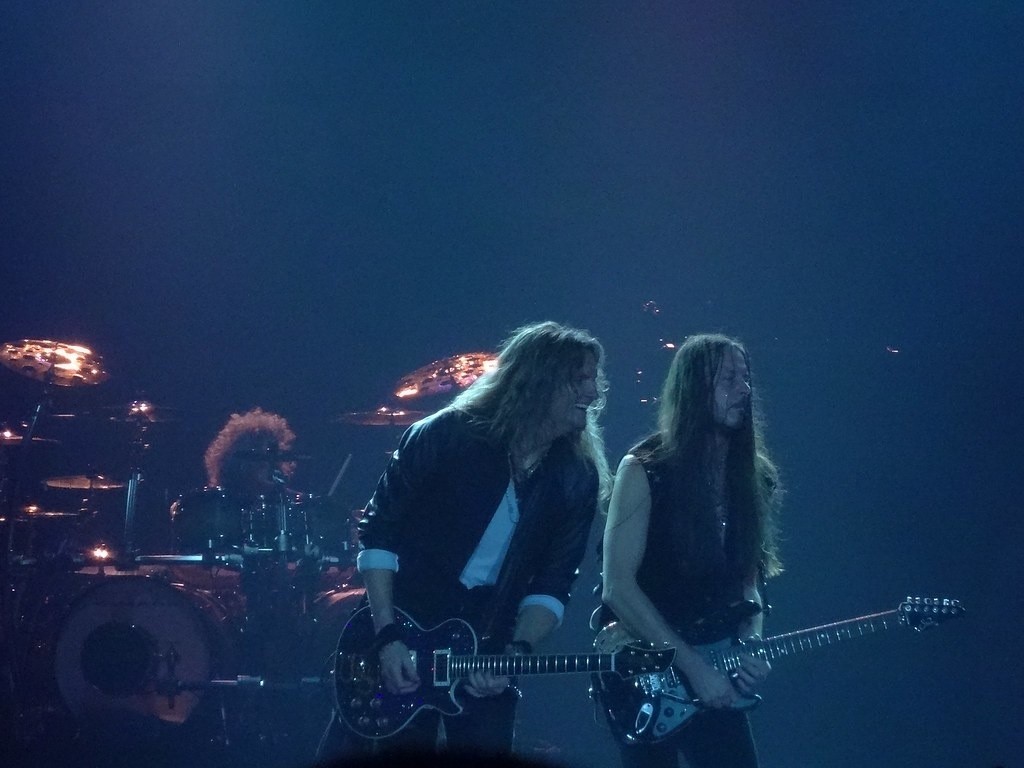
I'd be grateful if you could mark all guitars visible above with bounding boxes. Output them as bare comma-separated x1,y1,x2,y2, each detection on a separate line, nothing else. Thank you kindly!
592,595,967,746
334,603,678,741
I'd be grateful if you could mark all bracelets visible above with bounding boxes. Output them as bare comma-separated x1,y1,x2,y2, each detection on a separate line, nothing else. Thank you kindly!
508,640,533,654
372,625,401,652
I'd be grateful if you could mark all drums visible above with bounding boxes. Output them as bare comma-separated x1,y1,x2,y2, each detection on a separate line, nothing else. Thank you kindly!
167,486,245,589
240,490,328,561
1,569,217,735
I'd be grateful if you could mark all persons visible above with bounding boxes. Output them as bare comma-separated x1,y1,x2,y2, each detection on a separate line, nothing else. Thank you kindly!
172,407,318,556
65,622,201,768
590,333,786,768
309,322,605,768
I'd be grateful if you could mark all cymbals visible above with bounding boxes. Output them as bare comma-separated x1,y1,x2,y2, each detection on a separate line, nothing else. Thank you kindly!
0,338,109,387
87,403,182,423
242,449,312,462
329,405,437,427
0,430,58,445
393,352,496,400
1,508,79,520
38,474,124,489
